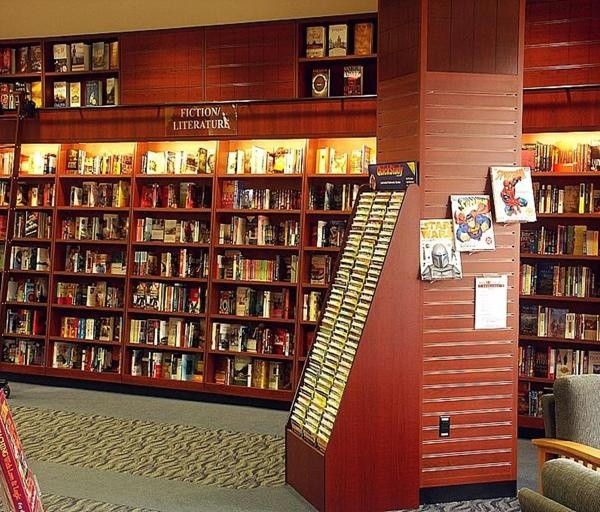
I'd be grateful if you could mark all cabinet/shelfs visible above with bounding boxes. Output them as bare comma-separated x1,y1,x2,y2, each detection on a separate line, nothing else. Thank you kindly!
0,138,58,378
0,35,121,112
295,134,379,403
281,159,425,511
519,136,599,431
123,137,218,395
48,139,135,384
207,134,306,401
294,16,379,98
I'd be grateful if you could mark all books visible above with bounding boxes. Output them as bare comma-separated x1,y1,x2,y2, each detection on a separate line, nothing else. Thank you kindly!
291,190,404,446
303,146,375,322
211,146,301,390
127,147,213,383
307,23,373,99
450,195,495,250
1,152,56,366
1,45,44,110
419,219,462,280
518,141,600,417
52,41,119,108
0,395,44,511
52,148,133,374
491,166,538,222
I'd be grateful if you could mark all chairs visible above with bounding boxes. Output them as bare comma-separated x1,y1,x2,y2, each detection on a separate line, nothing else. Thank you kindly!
517,372,600,512
